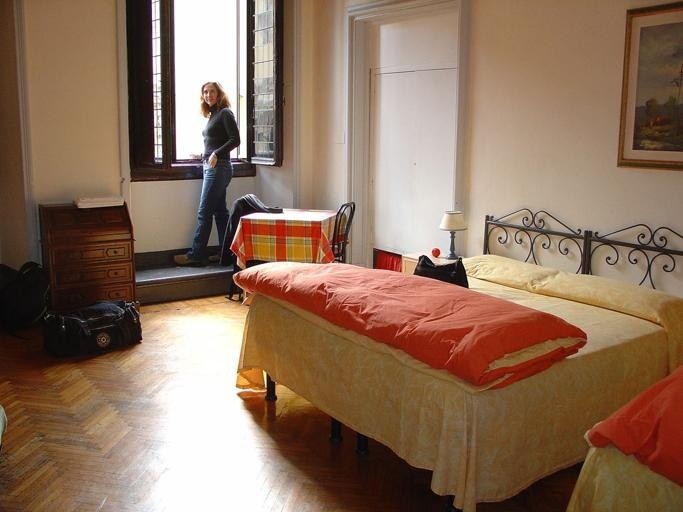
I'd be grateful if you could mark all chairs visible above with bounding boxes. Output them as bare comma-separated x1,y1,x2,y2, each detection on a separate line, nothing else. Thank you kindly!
227,193,255,302
332,202,356,264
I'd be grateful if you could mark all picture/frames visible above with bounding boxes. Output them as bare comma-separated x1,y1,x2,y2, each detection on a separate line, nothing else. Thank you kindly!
617,1,683,172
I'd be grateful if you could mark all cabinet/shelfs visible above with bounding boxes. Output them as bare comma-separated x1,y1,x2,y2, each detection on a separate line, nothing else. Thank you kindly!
37,200,137,314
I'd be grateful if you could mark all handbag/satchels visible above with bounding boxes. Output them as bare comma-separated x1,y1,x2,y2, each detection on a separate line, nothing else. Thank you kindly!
413,255,467,288
0,262,50,330
43,300,141,358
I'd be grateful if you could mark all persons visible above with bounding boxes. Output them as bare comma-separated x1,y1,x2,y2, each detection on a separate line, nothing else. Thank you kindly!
174,81,240,267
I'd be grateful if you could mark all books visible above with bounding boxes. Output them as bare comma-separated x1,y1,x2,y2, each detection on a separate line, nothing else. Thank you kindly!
73,195,124,208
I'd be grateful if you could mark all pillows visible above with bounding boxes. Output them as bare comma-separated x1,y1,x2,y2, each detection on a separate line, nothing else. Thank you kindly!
541,273,682,328
462,252,555,297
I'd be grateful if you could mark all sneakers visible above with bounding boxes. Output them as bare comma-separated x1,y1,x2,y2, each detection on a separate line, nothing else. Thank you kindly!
173,255,200,266
208,255,220,262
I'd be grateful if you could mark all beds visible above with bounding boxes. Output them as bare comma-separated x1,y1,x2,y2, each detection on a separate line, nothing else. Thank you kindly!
232,208,683,512
565,365,683,511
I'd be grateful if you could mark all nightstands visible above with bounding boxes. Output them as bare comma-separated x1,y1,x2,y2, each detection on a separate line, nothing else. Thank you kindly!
400,252,464,278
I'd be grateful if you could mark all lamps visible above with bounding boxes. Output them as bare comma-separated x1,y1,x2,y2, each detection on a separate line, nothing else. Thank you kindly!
440,210,468,259
